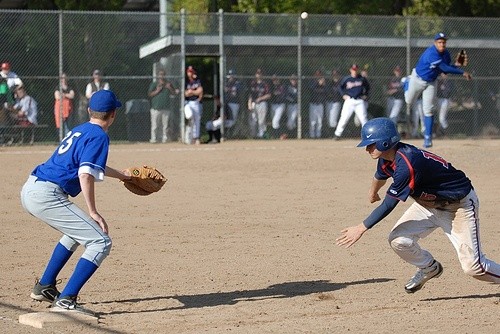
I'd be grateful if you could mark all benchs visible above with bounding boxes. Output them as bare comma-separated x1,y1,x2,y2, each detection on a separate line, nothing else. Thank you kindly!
1,123,50,145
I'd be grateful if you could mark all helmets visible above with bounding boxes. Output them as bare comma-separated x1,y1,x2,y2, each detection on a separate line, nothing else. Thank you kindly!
356,117,401,152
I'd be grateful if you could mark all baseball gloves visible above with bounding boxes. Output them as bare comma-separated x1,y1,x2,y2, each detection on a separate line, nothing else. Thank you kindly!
455,49,468,67
124,165,168,196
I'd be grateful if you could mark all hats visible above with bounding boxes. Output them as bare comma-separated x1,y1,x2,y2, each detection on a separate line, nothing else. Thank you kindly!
186,65,196,72
349,64,359,70
1,62,11,70
89,89,122,112
433,32,447,40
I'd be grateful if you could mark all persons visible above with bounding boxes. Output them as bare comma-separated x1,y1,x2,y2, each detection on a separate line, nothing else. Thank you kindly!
0,80,8,141
6,86,39,143
205,96,235,143
224,69,241,136
246,73,271,137
54,73,75,137
184,68,203,145
20,90,130,316
334,65,371,138
413,72,450,129
85,70,110,110
336,117,500,292
307,70,325,137
0,64,23,91
324,70,342,133
354,64,371,129
285,74,300,136
270,74,287,137
383,64,406,130
402,32,473,145
148,70,175,142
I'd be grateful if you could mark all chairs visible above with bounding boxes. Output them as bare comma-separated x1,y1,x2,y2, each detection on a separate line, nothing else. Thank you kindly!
224,103,239,138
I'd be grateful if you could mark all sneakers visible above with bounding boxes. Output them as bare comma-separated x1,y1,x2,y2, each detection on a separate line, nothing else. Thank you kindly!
30,277,62,303
51,293,95,316
404,259,443,293
425,134,431,147
